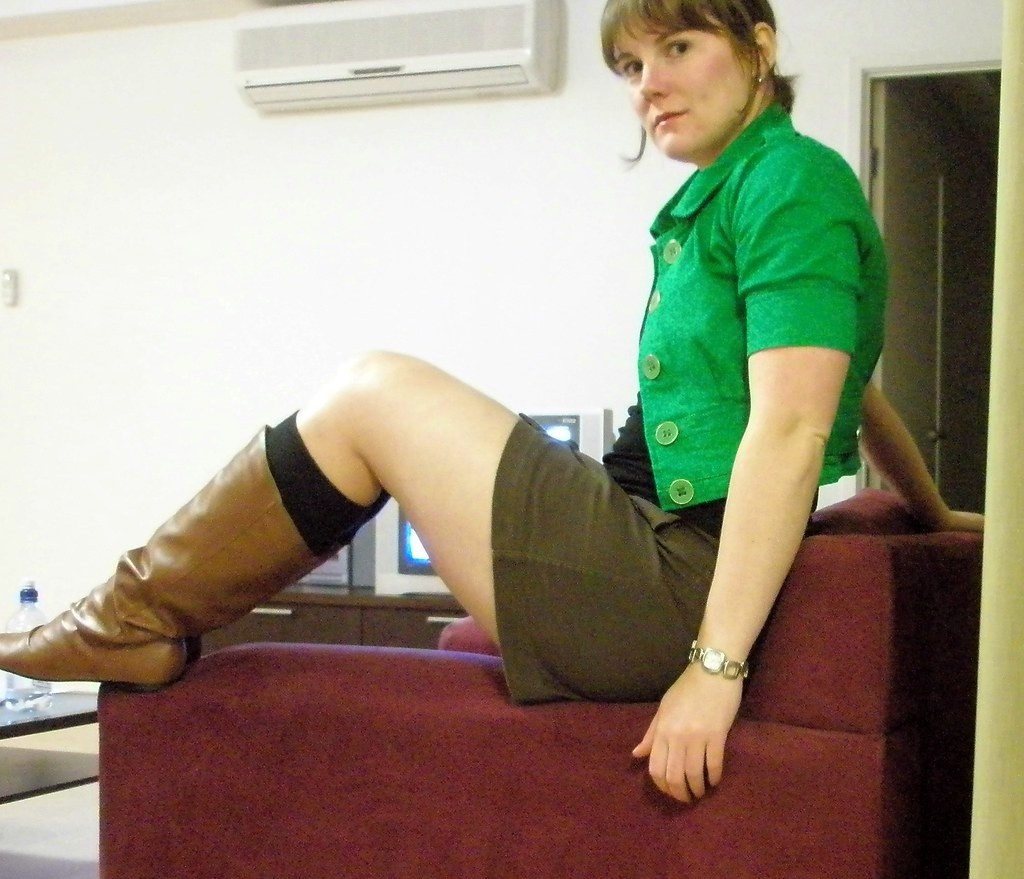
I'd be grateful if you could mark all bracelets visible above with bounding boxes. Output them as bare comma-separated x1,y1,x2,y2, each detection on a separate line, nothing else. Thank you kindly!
688,640,749,679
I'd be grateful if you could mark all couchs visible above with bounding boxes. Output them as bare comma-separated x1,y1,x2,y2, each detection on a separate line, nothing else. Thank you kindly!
97,487,984,879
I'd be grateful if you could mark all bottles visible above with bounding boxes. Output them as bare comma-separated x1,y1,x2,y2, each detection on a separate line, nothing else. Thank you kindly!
4,589,52,711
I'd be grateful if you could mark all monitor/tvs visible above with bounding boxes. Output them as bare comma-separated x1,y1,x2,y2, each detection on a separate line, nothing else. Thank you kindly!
373,409,618,597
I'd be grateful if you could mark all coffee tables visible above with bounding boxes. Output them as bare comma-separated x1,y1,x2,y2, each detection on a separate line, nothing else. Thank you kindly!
0,691,101,807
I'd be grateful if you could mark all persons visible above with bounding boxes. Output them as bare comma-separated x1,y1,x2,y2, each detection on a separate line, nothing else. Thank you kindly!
0,1,985,804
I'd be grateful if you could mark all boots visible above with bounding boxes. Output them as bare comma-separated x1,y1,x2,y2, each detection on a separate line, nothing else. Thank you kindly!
0,424,335,689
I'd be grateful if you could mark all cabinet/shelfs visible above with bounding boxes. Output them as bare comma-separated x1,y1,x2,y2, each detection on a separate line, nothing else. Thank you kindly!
199,574,470,656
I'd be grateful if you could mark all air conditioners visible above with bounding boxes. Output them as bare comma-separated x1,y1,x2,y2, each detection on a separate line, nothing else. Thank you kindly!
238,0,562,113
